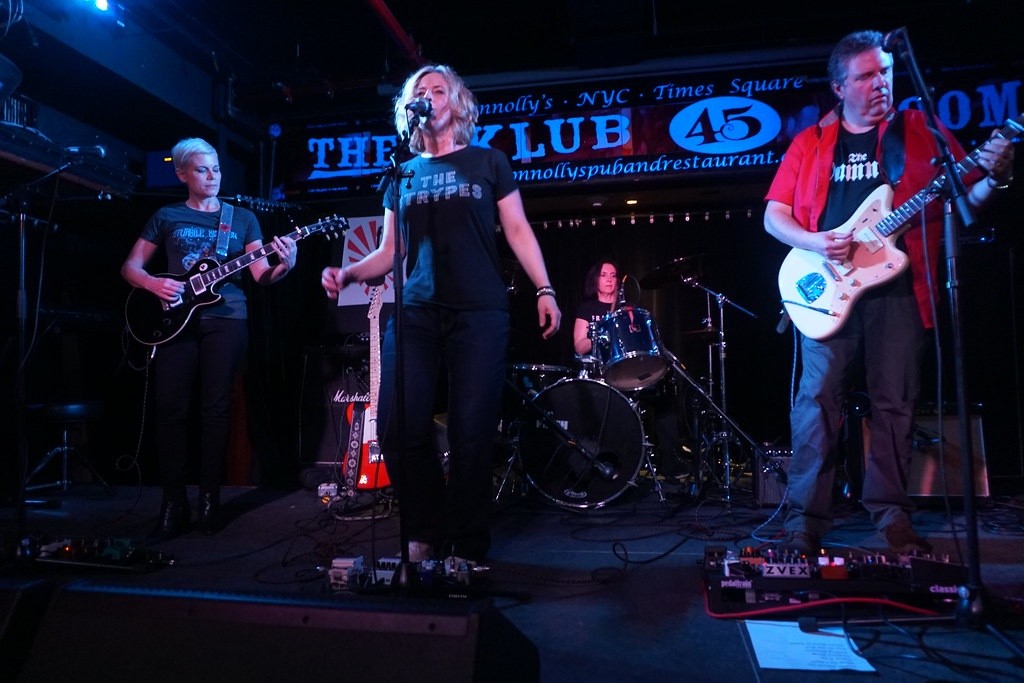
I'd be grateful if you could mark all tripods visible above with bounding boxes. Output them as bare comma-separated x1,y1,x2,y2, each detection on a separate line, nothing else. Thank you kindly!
681,274,757,504
799,34,1024,671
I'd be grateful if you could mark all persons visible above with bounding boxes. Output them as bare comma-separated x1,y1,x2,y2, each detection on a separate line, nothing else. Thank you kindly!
763,31,1015,557
572,259,631,376
321,64,562,603
121,137,297,547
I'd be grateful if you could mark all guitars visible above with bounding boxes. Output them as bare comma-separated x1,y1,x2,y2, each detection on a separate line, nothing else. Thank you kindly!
123,213,351,342
778,120,1024,342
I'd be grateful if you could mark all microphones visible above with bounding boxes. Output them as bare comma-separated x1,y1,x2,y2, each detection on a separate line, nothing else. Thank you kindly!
617,277,628,307
598,462,615,480
346,365,369,375
913,436,946,449
881,27,907,53
407,99,430,113
65,144,107,160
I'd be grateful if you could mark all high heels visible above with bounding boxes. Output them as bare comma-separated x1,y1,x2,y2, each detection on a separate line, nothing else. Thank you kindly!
144,502,192,545
199,497,225,536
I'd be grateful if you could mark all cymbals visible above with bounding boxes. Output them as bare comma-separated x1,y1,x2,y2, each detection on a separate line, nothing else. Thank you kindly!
496,258,529,280
680,327,719,333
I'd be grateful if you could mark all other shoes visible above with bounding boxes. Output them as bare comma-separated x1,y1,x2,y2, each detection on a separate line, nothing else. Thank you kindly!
438,563,480,608
391,561,426,601
790,532,825,562
880,513,932,555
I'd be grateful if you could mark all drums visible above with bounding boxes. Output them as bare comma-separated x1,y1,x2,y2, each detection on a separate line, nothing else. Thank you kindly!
509,370,666,513
593,306,669,391
513,364,579,397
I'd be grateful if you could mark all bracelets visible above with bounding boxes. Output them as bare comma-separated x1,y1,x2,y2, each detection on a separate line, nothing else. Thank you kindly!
987,175,1013,190
536,286,556,299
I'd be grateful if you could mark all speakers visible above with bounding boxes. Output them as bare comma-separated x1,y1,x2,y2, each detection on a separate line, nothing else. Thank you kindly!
844,405,993,512
297,347,370,469
752,446,796,510
0,579,52,683
20,581,543,683
